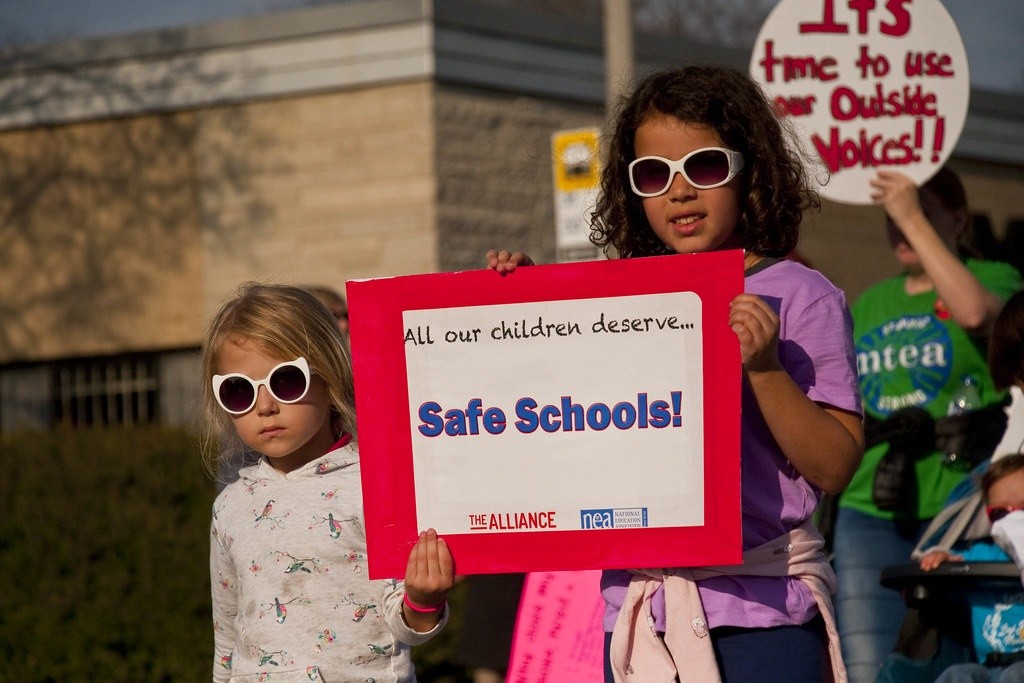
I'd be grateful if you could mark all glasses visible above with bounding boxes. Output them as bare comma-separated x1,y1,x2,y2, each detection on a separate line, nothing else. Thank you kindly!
211,356,317,415
629,148,744,199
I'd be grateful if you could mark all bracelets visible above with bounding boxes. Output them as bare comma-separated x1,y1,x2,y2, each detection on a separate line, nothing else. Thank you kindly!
404,595,447,613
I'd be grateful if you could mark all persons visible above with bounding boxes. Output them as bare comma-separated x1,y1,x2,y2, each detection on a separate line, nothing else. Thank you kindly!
485,63,865,683
833,167,1024,683
302,286,348,341
197,285,456,683
